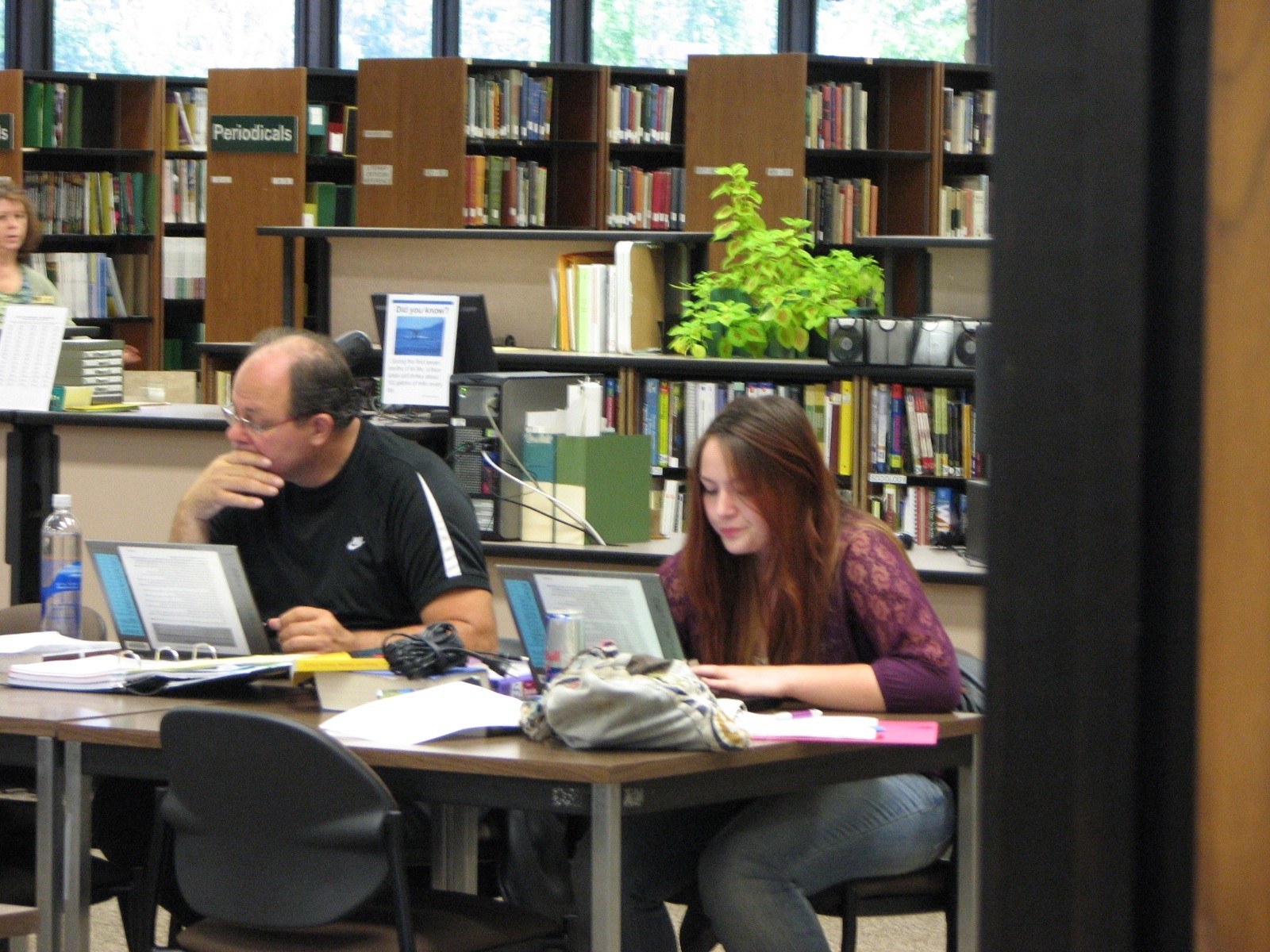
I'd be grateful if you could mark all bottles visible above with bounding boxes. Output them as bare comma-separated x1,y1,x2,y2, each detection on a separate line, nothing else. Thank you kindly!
41,494,82,642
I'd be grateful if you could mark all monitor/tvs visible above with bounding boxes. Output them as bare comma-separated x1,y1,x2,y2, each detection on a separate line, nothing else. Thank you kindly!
372,293,500,374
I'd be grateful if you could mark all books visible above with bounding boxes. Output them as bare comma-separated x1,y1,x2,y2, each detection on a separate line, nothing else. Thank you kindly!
801,174,878,244
567,369,980,548
22,78,123,148
805,77,868,152
545,241,689,356
199,353,238,405
0,629,540,740
939,175,990,240
161,85,207,300
461,69,554,142
943,87,995,155
607,82,676,146
802,255,884,357
23,172,157,234
714,698,939,746
606,159,687,230
22,252,149,318
466,153,548,227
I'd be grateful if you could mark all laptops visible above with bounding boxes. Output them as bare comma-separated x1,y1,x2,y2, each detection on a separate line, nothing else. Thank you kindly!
494,563,797,712
89,539,282,658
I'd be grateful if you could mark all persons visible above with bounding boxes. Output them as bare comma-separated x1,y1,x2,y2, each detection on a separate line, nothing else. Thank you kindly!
571,395,963,952
93,328,501,928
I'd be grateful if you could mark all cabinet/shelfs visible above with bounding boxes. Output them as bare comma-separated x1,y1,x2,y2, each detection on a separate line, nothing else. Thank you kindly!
0,52,994,659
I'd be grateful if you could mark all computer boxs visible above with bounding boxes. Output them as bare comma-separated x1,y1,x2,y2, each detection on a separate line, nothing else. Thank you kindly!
449,371,606,541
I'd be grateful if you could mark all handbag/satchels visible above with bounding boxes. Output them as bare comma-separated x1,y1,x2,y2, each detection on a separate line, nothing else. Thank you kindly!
519,640,749,755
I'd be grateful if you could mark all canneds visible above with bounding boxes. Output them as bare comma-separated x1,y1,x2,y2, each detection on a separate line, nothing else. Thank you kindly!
543,610,584,684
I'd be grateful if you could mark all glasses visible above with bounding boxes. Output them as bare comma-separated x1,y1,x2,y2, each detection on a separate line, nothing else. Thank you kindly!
221,401,320,433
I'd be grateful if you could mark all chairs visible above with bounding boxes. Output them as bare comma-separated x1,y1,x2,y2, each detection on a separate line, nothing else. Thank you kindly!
815,646,982,952
159,708,544,952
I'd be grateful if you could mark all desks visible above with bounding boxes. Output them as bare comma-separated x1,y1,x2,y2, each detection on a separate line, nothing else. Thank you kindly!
0,638,980,952
